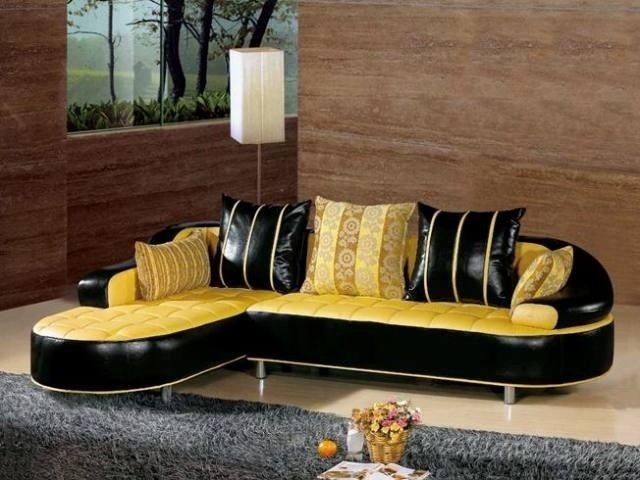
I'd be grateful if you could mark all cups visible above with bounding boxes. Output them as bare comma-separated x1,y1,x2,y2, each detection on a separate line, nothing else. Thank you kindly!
348,422,363,461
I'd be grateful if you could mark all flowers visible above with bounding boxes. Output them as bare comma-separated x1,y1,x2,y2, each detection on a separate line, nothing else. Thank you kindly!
352,398,423,435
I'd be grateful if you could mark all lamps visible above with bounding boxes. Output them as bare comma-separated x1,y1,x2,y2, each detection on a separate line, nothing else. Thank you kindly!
229,47,286,208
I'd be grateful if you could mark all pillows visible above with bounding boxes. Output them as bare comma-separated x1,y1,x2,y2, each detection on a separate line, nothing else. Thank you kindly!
403,201,526,308
212,191,312,294
300,195,418,303
510,245,574,312
134,228,212,303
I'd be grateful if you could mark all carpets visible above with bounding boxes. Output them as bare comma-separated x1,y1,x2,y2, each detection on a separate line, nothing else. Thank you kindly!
1,372,640,480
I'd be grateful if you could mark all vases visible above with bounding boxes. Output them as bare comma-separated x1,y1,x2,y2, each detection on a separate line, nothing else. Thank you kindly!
363,428,411,465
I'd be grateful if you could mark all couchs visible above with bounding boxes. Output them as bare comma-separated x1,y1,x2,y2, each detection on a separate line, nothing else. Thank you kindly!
30,222,614,407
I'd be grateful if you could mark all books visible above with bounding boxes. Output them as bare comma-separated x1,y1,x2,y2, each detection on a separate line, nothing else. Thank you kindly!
316,460,431,480
